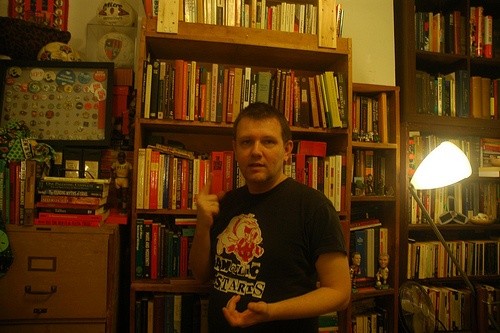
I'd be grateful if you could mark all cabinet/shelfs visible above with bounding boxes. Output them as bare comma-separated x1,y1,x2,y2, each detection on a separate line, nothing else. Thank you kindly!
401,122,500,333
393,0,500,128
129,16,353,333
0,223,122,333
352,82,402,329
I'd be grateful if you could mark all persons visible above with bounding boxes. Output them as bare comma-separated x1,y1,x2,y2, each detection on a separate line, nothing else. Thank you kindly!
189,102,352,332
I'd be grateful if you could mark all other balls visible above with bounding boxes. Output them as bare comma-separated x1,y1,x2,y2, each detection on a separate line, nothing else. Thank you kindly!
37,41,77,62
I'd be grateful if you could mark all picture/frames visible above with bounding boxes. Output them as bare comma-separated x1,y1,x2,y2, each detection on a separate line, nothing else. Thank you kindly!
0,60,114,147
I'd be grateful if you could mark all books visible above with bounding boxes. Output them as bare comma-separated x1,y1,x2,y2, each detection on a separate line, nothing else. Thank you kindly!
133,1,496,332
2,146,130,228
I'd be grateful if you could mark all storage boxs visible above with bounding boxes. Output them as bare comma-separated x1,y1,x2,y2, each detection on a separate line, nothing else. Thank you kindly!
112,69,133,86
84,23,138,69
112,86,129,117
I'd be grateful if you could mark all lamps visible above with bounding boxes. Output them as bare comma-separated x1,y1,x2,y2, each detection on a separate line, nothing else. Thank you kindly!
408,141,500,333
